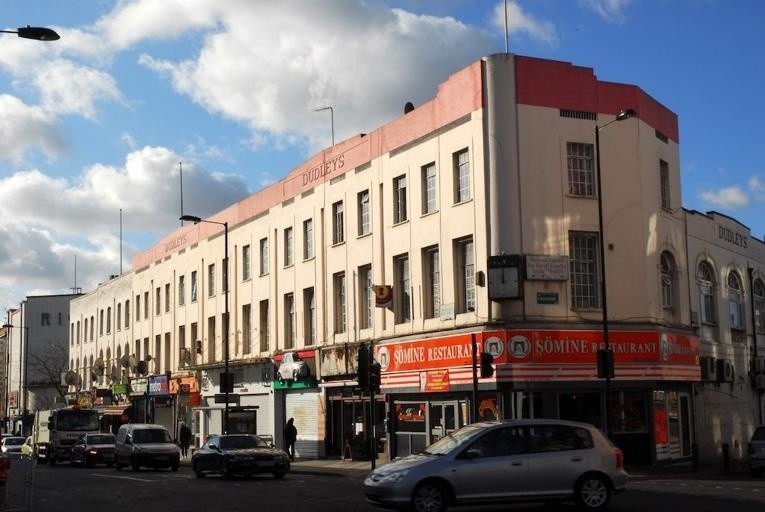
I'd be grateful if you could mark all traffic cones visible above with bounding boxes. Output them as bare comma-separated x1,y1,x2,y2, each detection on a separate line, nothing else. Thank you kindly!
732,441,742,471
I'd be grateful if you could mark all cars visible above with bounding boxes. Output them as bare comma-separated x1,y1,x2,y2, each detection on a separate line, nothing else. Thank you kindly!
191,434,291,479
276,353,308,385
364,417,628,510
748,425,765,468
72,432,116,467
1,434,33,461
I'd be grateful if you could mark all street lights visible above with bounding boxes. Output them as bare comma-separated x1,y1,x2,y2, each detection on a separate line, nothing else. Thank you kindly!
179,214,230,434
3,324,28,414
594,109,637,441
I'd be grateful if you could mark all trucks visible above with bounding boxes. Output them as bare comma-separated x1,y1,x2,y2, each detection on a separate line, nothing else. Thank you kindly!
32,408,101,465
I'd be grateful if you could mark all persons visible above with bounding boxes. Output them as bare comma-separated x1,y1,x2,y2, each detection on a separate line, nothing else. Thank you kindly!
180,421,191,460
490,344,498,355
662,341,668,356
285,417,297,462
514,343,526,356
381,355,387,368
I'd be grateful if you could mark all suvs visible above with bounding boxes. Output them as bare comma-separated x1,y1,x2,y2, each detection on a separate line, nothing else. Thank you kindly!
115,424,180,471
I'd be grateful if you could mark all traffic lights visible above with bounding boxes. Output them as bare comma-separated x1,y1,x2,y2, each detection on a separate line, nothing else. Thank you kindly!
479,352,495,380
369,363,383,384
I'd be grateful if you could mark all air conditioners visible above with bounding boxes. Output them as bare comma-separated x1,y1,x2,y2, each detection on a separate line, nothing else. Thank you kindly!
700,355,734,383
